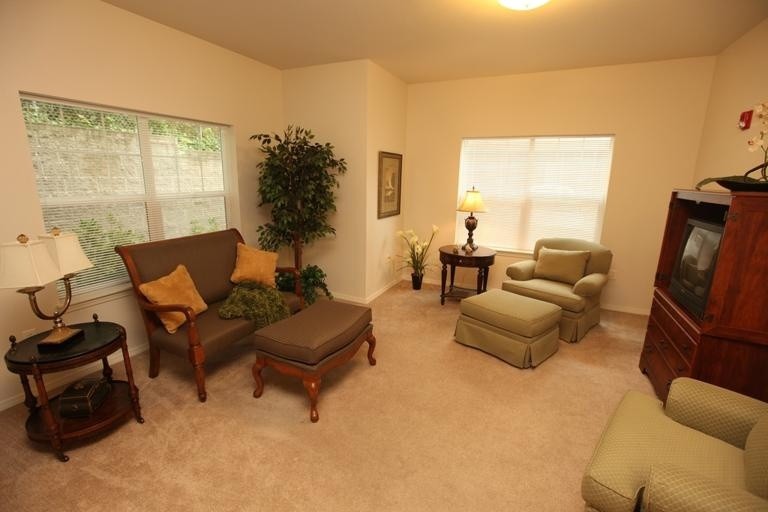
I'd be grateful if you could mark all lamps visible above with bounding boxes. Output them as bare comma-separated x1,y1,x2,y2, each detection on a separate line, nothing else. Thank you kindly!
454,187,490,251
1,224,98,356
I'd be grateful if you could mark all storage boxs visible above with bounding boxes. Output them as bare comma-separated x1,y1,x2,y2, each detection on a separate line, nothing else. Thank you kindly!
56,375,110,420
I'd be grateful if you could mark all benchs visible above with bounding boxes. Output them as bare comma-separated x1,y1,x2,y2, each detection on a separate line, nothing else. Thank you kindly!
247,295,379,426
112,228,301,403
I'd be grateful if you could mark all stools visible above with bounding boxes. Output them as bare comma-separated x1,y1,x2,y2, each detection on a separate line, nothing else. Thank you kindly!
452,285,564,370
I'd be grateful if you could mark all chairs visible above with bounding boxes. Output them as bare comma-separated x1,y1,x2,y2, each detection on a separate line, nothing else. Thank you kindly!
579,375,767,512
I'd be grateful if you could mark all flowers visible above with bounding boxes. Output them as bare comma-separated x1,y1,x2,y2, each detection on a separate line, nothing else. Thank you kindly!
736,103,767,183
387,221,445,274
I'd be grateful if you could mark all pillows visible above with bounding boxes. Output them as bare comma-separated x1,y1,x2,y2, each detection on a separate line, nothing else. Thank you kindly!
135,262,211,336
228,240,281,291
532,243,594,285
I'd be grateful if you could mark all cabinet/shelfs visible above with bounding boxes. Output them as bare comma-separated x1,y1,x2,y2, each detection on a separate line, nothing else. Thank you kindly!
636,186,767,413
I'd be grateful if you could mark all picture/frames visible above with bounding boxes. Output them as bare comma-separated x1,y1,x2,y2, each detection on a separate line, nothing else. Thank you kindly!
375,150,404,222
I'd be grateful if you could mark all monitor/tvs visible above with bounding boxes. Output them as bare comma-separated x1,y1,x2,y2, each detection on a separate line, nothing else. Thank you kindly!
668,217,724,319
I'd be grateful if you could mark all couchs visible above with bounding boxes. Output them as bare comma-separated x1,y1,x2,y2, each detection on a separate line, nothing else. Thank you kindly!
500,234,615,346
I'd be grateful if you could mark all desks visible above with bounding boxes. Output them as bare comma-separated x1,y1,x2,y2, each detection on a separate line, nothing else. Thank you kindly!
4,318,148,464
438,243,498,308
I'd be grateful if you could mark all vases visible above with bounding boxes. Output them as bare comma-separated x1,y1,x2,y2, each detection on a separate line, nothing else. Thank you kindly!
698,172,764,193
410,271,425,291
